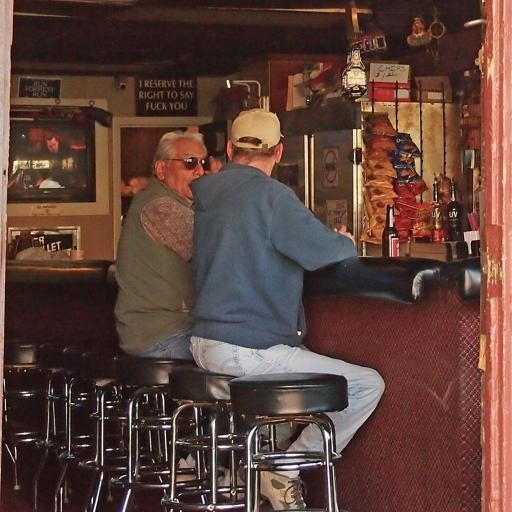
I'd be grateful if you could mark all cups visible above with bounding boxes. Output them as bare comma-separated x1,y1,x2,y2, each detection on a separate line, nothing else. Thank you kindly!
70,250,86,261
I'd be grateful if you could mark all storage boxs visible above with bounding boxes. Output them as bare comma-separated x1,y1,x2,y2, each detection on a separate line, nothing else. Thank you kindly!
370,62,410,83
368,82,411,102
415,76,453,103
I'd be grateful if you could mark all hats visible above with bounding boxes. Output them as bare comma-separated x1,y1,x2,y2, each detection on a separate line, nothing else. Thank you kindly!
231,108,284,149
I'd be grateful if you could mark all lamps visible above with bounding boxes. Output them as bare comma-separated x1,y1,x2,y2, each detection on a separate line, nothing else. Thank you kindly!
229,80,267,108
343,42,366,102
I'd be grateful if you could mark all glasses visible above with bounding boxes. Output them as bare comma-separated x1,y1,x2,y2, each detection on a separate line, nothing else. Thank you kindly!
164,156,209,170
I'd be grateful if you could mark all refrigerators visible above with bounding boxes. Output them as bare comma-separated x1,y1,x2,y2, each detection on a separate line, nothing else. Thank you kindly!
275,109,361,251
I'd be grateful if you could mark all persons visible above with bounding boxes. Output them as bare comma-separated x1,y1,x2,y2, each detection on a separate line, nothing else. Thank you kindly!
186,109,386,512
33,134,74,189
112,129,257,501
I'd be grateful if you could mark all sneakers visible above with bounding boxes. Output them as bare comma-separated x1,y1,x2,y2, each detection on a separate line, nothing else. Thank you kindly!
217,469,246,500
177,457,207,490
259,472,306,511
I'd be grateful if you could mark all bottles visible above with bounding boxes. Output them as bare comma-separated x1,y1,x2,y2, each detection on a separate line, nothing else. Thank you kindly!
382,205,399,258
430,181,444,243
446,180,463,239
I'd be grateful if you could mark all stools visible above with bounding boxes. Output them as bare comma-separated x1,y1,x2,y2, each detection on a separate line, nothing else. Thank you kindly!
162,362,248,511
4,339,112,512
231,374,348,512
111,354,197,512
